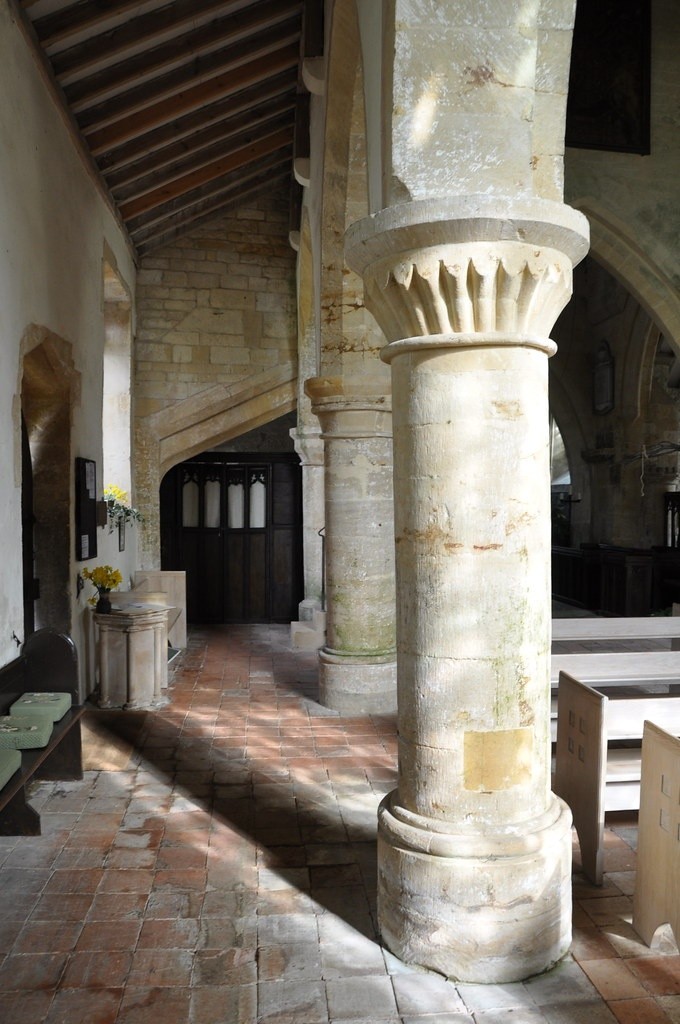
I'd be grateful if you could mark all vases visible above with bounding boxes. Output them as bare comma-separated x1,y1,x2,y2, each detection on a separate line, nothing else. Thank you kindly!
108,501,114,506
96,588,112,614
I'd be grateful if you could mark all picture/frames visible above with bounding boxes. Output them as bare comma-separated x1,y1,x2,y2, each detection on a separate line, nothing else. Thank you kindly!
118,516,125,551
75,456,98,562
593,342,615,416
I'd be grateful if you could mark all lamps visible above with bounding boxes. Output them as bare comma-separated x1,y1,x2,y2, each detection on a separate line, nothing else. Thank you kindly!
559,484,582,550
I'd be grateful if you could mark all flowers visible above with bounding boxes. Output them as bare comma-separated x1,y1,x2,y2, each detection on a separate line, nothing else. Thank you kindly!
104,485,128,503
82,564,123,606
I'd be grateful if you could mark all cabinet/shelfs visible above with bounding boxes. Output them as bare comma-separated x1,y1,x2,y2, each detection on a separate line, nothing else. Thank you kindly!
630,718,680,954
554,669,680,888
551,542,680,617
550,602,680,744
0,627,86,840
134,570,187,649
